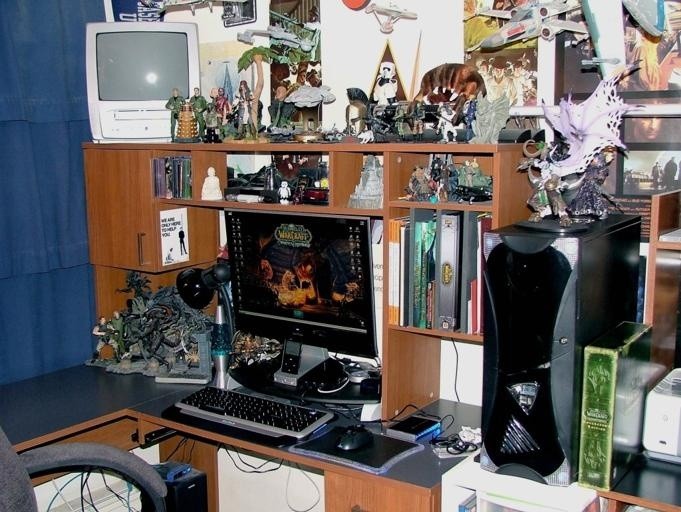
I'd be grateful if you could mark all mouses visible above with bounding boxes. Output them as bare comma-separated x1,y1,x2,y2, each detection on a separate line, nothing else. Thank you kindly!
331,424,374,453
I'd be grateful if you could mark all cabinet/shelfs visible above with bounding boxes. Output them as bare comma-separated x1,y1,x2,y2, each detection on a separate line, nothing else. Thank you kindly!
600,186,681,512
84,142,225,358
223,143,543,424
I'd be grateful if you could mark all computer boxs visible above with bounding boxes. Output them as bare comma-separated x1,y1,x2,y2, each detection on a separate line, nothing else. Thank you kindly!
481,214,641,487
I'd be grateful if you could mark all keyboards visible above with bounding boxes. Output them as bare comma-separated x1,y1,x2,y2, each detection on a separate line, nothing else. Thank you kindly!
174,384,334,439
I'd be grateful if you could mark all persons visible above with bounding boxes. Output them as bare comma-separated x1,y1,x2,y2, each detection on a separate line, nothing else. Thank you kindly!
410,161,476,202
202,168,222,200
663,155,679,190
625,161,662,188
528,154,609,227
279,182,290,204
629,98,667,143
268,88,298,127
363,102,477,143
179,226,189,255
166,80,256,143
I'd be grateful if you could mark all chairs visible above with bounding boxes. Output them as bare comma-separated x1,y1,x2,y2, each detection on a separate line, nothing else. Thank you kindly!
0,425,168,511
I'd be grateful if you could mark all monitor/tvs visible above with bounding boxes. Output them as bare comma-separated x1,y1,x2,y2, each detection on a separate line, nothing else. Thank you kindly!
85,21,202,145
224,206,381,404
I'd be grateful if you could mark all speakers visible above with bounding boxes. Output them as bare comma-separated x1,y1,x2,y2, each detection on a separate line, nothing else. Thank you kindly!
140,461,208,512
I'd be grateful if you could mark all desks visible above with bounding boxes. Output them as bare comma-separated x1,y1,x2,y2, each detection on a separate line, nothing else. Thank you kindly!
3,367,205,488
126,386,479,512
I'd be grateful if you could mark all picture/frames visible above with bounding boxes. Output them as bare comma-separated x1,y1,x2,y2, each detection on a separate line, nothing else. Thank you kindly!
553,0,681,197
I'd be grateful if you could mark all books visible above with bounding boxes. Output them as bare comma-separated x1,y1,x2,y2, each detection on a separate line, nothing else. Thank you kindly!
459,491,478,511
385,210,487,340
465,498,478,512
227,330,285,371
152,158,191,199
